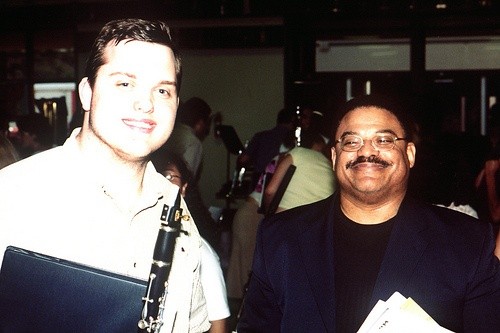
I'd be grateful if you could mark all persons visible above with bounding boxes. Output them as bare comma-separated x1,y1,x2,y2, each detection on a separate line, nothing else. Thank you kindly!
265,139,336,216
0,99,500,189
0,19,231,333
233,94,500,333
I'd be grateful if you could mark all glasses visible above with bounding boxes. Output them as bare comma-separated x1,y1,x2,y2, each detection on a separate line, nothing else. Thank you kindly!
163,172,183,181
334,135,410,152
